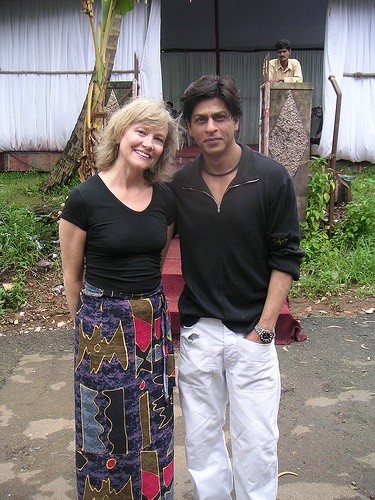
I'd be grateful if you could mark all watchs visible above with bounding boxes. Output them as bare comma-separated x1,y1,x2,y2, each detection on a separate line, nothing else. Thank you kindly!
254,322,276,344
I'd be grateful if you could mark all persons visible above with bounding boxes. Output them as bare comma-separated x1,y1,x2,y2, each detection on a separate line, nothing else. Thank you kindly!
56,74,306,500
263,38,303,83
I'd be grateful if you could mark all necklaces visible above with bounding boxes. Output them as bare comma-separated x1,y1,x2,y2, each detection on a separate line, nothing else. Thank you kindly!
201,149,243,177
